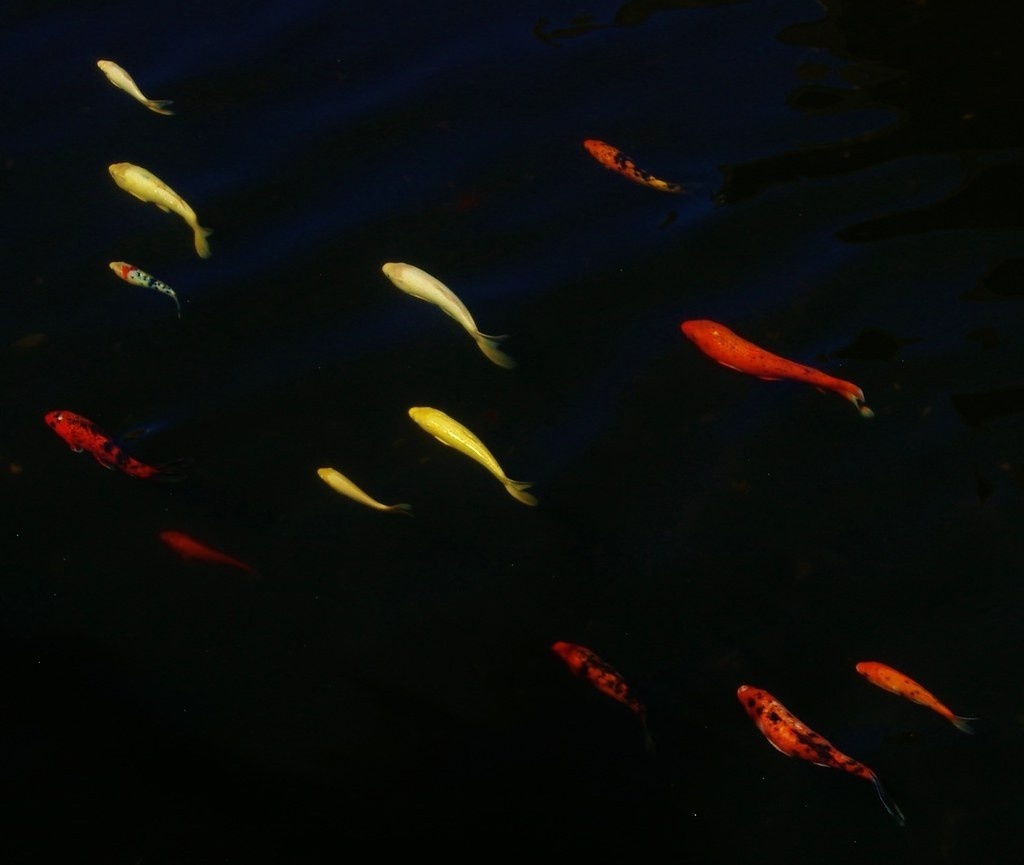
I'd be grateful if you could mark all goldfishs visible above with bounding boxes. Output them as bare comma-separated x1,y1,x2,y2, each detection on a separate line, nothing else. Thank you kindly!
38,59,978,825
